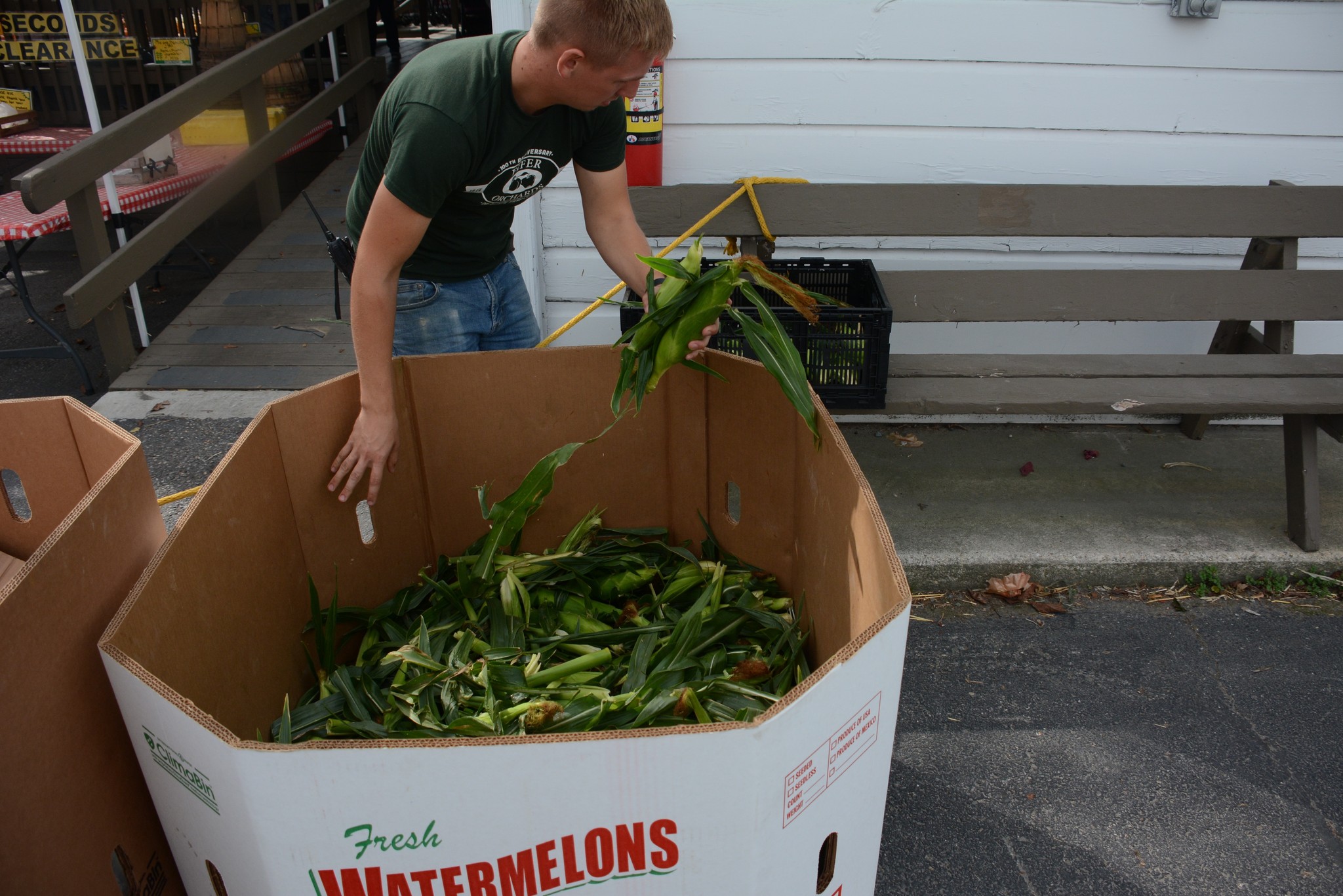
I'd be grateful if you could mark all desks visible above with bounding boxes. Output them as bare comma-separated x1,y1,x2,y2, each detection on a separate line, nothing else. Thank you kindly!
1,115,336,399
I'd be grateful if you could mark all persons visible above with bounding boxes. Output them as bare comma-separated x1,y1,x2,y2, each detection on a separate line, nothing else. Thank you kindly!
328,0,733,506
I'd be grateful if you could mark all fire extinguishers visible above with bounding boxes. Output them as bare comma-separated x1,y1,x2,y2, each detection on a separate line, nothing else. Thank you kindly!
625,32,677,187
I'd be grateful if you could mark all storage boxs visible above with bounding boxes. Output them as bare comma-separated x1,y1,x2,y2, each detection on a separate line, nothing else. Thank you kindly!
0,397,194,896
93,343,918,896
618,257,894,411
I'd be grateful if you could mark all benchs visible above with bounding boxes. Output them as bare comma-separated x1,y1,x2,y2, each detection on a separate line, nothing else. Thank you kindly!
617,180,1343,555
